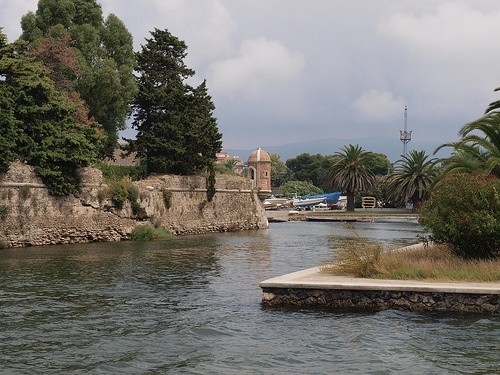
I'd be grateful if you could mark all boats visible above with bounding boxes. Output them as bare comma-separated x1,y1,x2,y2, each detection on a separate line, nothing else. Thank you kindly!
263,191,341,211
